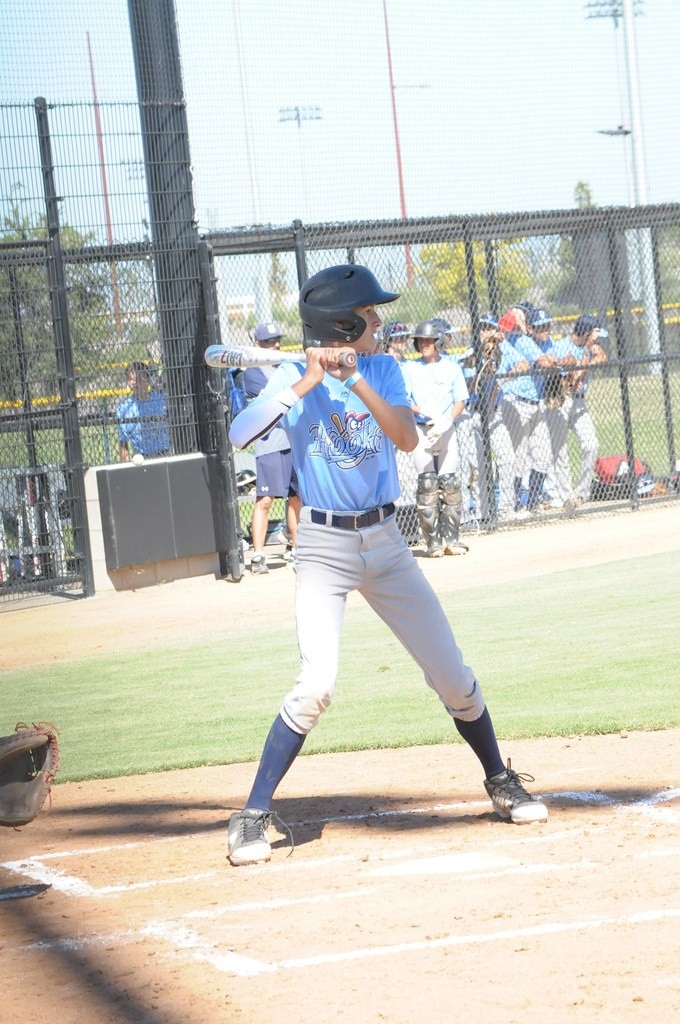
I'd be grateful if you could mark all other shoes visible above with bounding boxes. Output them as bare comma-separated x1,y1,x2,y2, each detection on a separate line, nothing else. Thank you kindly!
445,545,469,555
251,554,270,574
427,542,443,558
284,544,295,560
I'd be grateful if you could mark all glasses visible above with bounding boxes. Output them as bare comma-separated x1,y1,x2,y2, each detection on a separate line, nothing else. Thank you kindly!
263,336,281,343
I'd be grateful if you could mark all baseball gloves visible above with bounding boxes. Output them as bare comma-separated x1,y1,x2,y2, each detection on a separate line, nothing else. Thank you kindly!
0,721,59,826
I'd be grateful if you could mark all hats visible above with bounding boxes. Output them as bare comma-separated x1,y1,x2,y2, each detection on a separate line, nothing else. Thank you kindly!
576,313,609,338
477,300,554,334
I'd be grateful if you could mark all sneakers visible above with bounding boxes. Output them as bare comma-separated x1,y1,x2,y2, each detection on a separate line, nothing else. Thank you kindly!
227,807,294,865
484,758,549,824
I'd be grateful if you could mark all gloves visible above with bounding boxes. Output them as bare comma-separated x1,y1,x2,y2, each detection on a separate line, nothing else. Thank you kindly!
427,413,453,441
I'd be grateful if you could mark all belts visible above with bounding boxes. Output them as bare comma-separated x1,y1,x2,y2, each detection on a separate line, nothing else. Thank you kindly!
568,394,585,399
516,395,540,406
312,503,395,530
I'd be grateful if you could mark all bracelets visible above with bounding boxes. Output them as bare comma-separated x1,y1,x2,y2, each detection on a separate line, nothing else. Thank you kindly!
344,372,361,388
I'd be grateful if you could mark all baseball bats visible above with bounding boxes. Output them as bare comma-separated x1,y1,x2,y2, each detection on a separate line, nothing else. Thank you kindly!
203,344,356,368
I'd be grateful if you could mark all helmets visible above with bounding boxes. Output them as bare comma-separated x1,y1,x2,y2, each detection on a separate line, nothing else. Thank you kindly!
254,322,286,341
382,319,413,344
299,264,400,342
411,320,447,352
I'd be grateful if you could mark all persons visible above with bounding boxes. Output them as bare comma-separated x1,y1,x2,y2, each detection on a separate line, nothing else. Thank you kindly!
400,320,470,557
116,341,172,463
381,321,409,361
228,264,548,862
244,323,303,574
437,303,606,523
